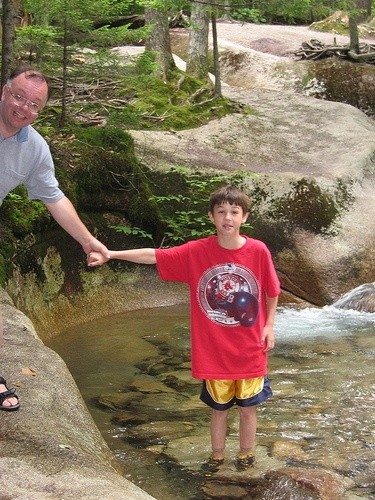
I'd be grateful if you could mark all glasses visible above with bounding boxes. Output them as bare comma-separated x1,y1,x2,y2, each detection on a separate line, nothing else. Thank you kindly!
8,86,43,115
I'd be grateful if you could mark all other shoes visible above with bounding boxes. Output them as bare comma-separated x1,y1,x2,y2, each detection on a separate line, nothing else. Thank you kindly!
198,457,225,476
236,452,256,471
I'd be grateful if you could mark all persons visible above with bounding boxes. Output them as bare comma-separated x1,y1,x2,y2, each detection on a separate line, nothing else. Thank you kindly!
0,70,111,411
86,186,281,473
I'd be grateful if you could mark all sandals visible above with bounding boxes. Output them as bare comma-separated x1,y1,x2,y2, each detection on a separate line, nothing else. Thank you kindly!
0,375,21,411
